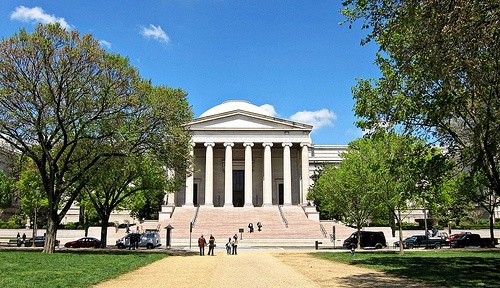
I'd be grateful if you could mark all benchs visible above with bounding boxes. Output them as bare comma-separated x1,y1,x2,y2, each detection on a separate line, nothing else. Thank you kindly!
8,240,21,245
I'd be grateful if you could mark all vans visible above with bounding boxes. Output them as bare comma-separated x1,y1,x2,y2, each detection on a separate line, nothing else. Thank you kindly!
343,231,387,249
445,232,471,246
114,233,162,249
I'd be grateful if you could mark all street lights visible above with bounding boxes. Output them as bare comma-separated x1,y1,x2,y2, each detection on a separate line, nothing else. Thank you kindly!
31,186,40,247
421,189,430,249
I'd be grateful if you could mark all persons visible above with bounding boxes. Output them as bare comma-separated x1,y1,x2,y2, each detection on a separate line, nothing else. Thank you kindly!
248,223,253,233
17,232,26,243
225,234,238,255
128,232,141,250
198,234,216,256
257,222,262,231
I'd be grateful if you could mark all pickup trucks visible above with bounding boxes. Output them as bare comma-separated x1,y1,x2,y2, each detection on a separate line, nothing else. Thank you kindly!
449,234,498,249
402,235,446,249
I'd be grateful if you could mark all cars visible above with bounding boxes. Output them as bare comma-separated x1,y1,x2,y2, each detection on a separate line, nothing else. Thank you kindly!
17,236,60,248
64,237,103,248
